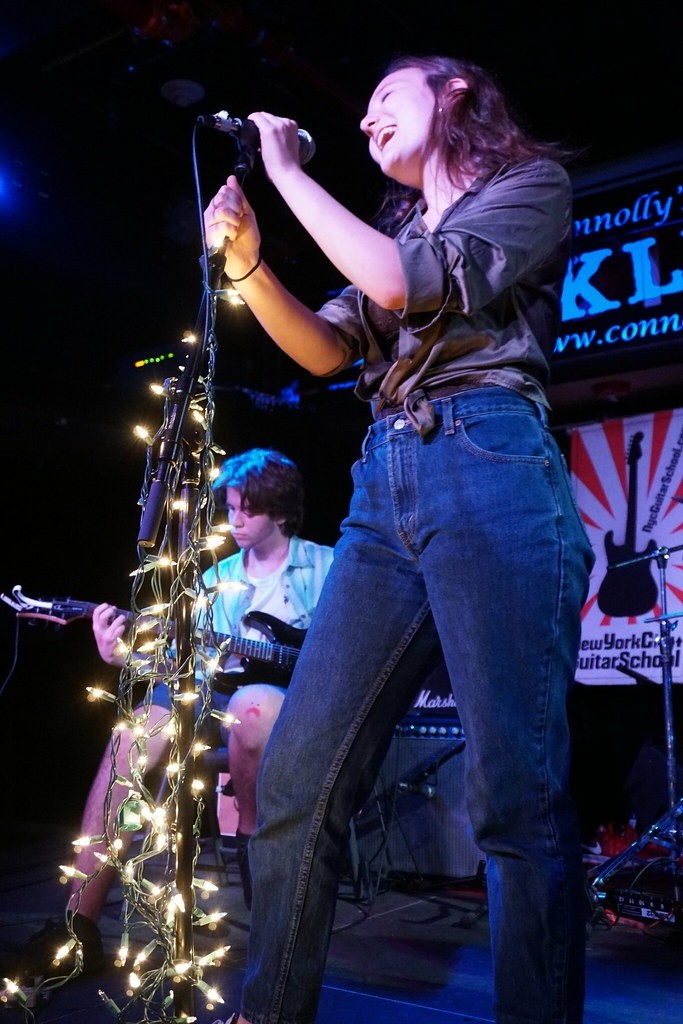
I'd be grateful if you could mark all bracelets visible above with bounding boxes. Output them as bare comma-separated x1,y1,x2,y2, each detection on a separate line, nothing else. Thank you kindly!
227,255,262,282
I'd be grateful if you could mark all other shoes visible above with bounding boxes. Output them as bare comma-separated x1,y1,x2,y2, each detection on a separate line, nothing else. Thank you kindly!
236,829,254,911
10,917,106,982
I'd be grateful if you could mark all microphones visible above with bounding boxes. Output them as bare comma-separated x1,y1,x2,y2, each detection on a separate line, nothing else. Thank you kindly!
199,112,315,166
397,781,434,798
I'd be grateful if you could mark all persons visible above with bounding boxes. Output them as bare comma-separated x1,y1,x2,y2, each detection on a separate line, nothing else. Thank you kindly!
0,449,335,988
203,54,598,1023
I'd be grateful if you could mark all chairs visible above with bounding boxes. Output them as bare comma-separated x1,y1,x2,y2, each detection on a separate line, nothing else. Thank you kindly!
156,743,368,904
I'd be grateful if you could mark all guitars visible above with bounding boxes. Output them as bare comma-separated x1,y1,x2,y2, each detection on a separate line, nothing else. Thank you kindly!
0,585,309,696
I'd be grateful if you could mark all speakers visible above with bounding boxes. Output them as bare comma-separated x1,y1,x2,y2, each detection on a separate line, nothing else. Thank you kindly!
348,713,490,887
